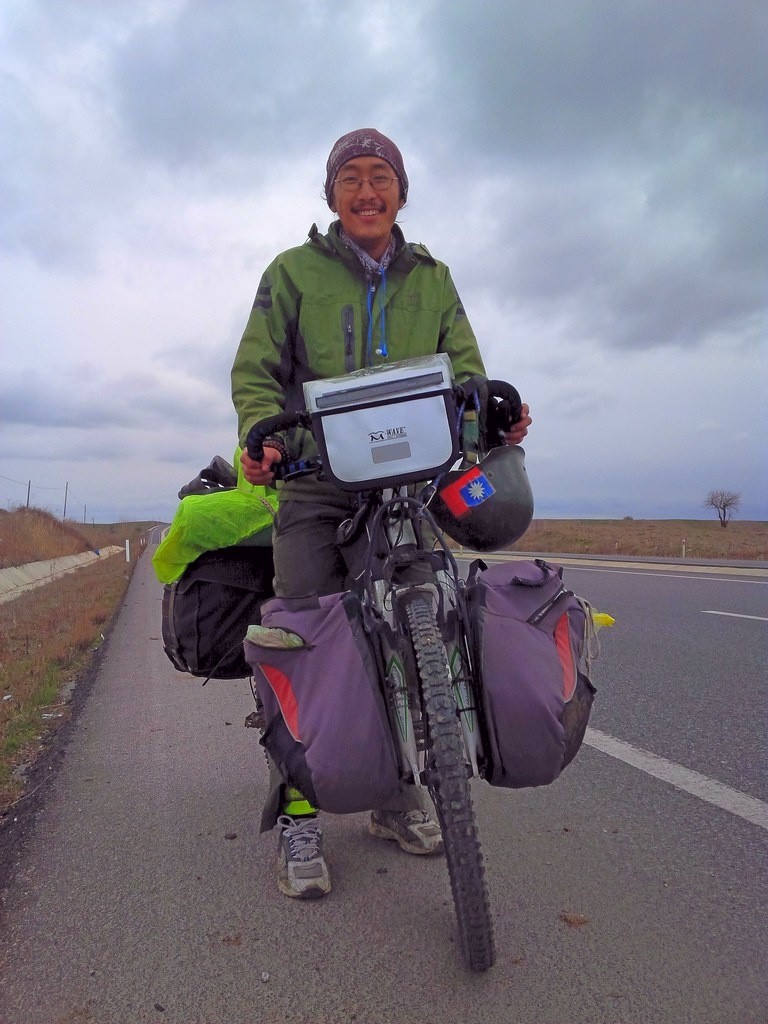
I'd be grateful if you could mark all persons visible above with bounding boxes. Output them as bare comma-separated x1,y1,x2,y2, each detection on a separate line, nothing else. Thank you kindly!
231,127,532,898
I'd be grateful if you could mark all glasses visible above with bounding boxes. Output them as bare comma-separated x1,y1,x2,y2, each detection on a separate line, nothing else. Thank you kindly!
332,173,398,191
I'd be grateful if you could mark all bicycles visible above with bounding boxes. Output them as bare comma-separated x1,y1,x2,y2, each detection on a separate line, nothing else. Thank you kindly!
246,379,523,974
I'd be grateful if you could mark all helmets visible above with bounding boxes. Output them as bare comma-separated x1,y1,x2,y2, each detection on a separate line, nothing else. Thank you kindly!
420,445,534,552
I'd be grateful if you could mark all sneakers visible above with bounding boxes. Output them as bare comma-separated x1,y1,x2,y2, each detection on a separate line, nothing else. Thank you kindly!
368,803,445,855
276,818,331,899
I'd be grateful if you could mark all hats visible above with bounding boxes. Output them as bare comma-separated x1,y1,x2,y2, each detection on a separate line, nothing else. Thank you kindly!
325,128,409,200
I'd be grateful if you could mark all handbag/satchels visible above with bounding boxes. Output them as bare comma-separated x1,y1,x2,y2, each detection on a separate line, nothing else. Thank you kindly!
467,556,612,786
161,494,275,686
466,558,615,788
243,592,403,814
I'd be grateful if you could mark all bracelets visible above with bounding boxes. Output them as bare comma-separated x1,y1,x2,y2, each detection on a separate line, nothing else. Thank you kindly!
263,440,290,465
